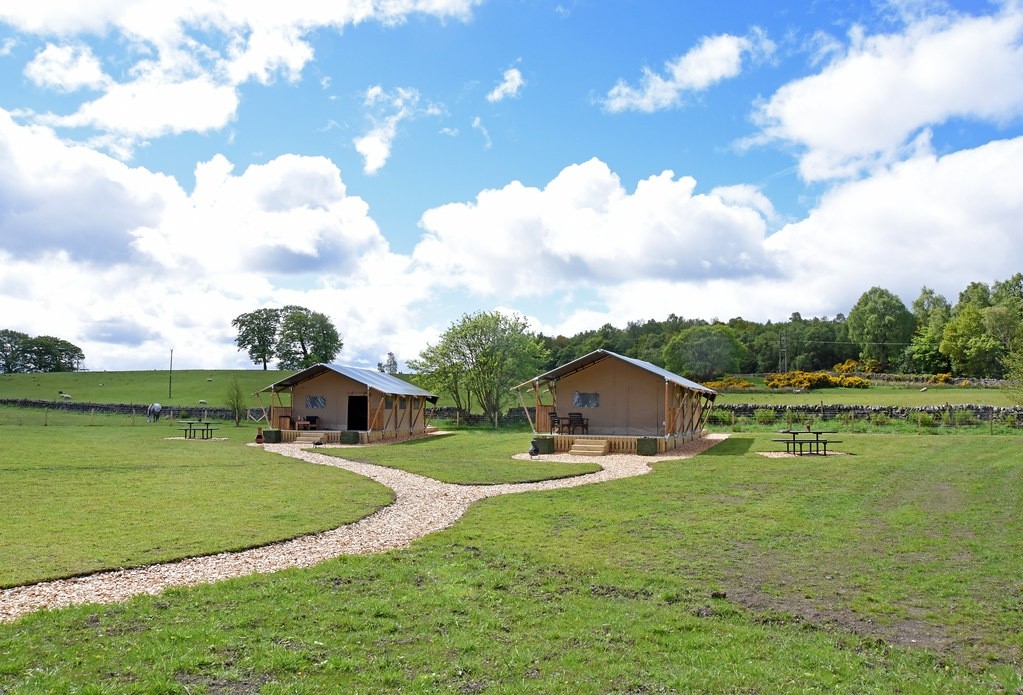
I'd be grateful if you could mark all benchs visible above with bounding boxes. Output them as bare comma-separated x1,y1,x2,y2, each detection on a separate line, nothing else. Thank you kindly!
771,439,842,456
177,428,219,439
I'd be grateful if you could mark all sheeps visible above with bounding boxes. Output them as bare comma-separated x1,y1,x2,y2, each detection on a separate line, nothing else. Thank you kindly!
58,390,71,399
146,403,161,422
199,400,207,404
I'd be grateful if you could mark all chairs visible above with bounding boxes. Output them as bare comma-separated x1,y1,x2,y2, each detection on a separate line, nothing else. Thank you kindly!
305,416,319,430
568,412,589,435
548,411,569,435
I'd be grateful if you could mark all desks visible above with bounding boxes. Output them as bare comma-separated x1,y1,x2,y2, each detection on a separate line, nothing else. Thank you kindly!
177,421,222,439
778,431,837,454
296,421,310,431
